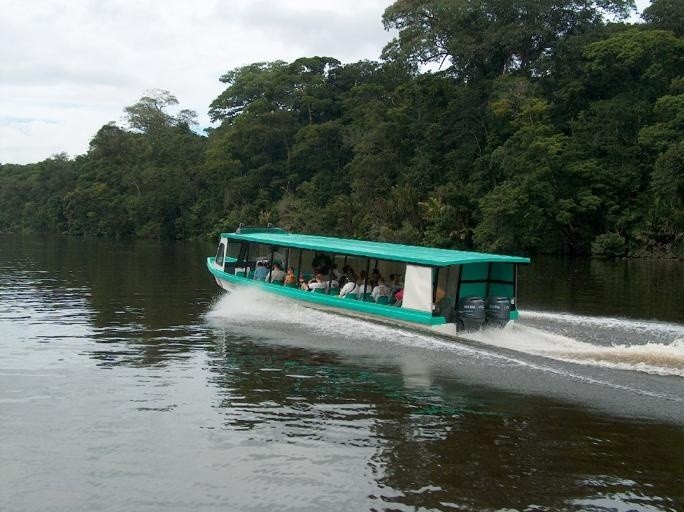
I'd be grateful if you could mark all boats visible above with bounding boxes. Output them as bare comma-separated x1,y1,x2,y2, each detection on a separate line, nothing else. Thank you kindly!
205,222,533,340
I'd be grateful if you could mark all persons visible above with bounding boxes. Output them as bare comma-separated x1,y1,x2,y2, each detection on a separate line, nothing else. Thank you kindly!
251,246,404,308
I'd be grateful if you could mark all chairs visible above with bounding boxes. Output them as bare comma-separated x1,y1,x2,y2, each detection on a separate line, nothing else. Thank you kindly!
235,266,401,307
